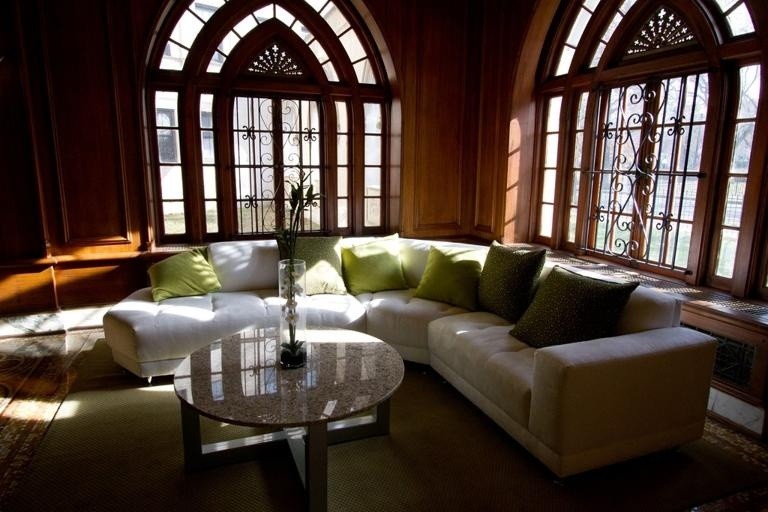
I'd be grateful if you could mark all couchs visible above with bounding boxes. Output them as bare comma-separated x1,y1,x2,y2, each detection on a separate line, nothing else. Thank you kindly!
103,286,717,478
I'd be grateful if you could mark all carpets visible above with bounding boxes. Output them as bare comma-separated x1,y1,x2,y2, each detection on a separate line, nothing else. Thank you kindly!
3,338,768,512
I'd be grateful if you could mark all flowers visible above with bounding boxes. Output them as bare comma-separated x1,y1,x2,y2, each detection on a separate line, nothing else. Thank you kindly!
278,165,327,355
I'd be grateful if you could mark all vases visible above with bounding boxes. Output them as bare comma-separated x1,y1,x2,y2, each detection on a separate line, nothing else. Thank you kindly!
278,259,306,369
